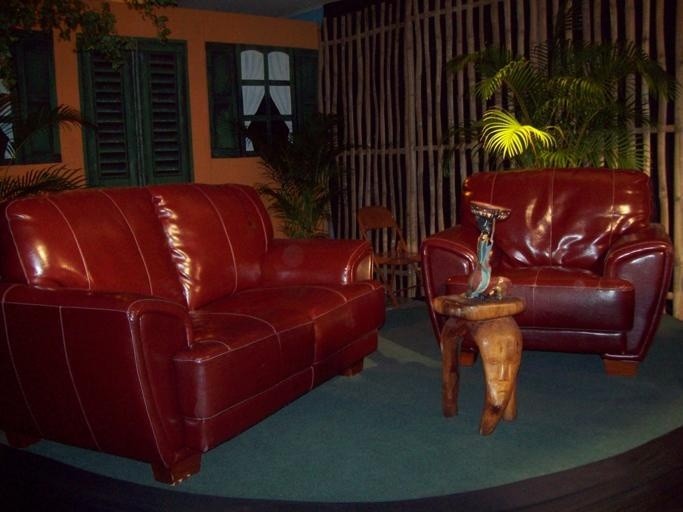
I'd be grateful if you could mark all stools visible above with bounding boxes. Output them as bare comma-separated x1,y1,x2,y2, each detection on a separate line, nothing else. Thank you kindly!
433,292,524,436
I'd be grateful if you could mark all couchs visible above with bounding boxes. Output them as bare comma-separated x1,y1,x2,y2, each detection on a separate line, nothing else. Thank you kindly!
420,168,673,377
0,184,389,485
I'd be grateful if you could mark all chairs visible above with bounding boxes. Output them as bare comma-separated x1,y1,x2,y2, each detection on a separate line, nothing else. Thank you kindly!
359,206,426,307
